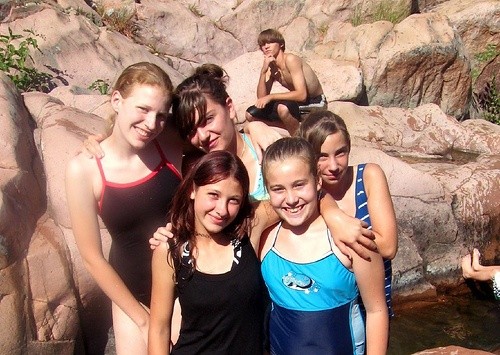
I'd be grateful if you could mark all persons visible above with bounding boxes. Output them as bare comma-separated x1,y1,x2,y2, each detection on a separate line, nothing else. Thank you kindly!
246,29,322,137
461,248,500,301
65,62,397,355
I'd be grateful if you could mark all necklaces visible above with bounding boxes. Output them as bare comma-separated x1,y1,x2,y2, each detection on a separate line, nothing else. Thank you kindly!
194,233,209,238
240,142,246,158
